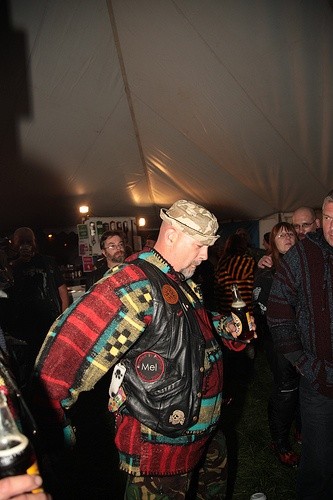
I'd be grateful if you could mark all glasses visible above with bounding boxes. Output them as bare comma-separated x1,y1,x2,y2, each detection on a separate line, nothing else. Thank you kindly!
276,233,296,239
102,244,125,250
291,219,316,230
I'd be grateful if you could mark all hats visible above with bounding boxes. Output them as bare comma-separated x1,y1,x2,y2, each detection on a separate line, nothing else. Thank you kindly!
11,227,35,244
160,199,220,246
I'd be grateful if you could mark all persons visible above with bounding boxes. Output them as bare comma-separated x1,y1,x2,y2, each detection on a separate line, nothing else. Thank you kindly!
195,197,333,500
0,227,70,336
80,232,131,284
32,200,257,500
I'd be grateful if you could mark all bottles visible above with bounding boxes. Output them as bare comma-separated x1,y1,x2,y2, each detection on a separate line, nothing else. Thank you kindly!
229,283,254,342
0,353,45,494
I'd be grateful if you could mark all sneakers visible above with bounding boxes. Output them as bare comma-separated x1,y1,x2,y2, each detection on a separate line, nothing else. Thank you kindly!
272,444,299,467
